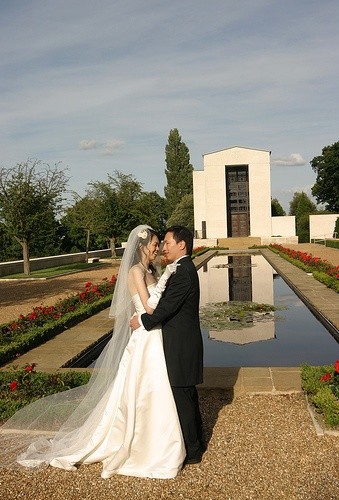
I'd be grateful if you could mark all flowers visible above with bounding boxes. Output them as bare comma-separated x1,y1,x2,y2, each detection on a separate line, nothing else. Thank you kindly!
137,231,148,238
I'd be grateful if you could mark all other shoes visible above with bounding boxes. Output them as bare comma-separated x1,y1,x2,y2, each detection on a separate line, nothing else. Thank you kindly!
199,445,206,452
184,453,201,464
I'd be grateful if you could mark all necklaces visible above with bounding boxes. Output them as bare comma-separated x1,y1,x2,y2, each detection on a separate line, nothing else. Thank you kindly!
139,262,152,273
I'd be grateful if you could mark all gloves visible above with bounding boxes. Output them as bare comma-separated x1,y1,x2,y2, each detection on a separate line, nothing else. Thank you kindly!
147,264,181,309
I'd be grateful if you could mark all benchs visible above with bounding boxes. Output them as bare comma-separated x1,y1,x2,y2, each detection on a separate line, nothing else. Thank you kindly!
85,257,100,263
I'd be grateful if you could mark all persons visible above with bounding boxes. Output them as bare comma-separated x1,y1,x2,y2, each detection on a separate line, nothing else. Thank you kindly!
0,224,186,480
128,224,206,465
